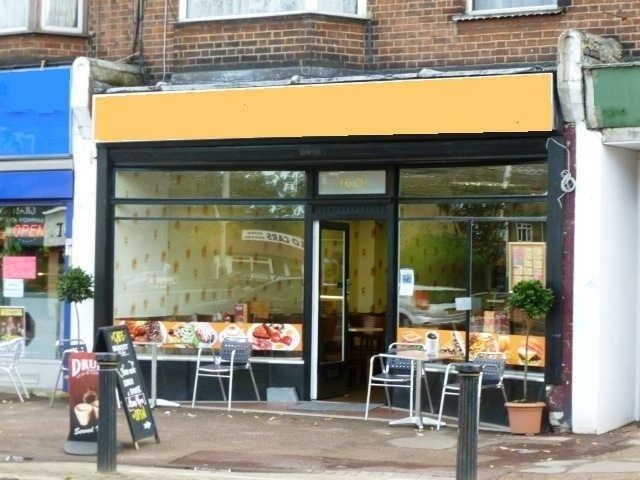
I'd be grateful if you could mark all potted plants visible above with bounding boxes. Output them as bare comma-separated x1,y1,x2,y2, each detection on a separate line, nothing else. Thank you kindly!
504,278,554,437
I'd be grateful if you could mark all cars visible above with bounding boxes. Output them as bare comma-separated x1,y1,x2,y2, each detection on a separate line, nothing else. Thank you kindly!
399,282,468,330
186,276,304,317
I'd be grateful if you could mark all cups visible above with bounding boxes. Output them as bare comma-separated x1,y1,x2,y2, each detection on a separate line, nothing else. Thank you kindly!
424,332,439,354
226,325,241,335
73,386,99,427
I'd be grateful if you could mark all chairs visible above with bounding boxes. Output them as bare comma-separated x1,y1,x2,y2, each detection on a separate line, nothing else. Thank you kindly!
191,337,260,408
50,338,121,410
1,337,30,402
364,342,507,433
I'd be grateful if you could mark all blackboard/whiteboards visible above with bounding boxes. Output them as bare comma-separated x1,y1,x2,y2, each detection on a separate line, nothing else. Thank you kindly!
100,324,157,441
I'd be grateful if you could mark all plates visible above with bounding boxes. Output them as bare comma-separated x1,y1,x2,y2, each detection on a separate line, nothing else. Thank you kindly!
219,329,245,345
120,320,167,347
168,322,217,348
248,323,300,351
